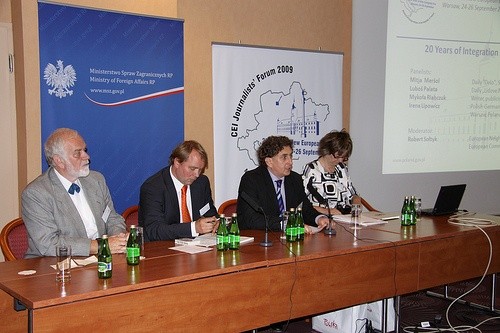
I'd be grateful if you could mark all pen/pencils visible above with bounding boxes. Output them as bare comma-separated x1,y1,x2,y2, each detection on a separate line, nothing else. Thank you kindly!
201,216,214,225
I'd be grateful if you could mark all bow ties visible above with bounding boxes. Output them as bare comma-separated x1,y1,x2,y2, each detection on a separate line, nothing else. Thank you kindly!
68,183,80,195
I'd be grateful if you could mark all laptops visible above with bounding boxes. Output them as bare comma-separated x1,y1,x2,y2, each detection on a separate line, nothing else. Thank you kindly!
415,185,464,215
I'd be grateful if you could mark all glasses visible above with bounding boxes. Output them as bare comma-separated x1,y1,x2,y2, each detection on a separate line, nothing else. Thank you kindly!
333,153,349,161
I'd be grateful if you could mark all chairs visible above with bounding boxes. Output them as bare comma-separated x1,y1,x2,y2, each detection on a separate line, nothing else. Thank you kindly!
122,205,138,229
0,218,29,262
218,199,239,219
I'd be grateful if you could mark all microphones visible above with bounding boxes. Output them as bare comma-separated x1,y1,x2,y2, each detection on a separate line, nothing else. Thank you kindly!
240,192,272,247
307,185,335,234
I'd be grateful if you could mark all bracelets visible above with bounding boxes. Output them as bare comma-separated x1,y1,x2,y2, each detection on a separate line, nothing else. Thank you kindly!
96,237,102,255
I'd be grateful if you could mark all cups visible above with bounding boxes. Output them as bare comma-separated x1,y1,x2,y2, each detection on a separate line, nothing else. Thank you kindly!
350,204,361,230
56,245,73,282
133,227,145,251
414,197,422,221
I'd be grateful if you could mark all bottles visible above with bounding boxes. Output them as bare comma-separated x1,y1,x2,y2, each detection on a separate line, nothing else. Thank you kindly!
98,234,113,279
126,225,141,265
401,196,412,226
228,212,240,250
216,214,229,250
296,208,305,241
285,208,298,242
409,195,417,224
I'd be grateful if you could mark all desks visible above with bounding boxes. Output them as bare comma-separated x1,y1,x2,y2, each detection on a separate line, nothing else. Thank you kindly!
0,205,500,333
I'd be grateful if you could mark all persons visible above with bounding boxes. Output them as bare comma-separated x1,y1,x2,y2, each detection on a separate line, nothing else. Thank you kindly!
21,128,129,259
302,131,361,217
237,136,335,333
137,140,224,243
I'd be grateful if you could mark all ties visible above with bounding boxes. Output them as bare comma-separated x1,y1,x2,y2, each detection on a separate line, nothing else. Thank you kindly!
276,180,285,214
181,185,191,223
